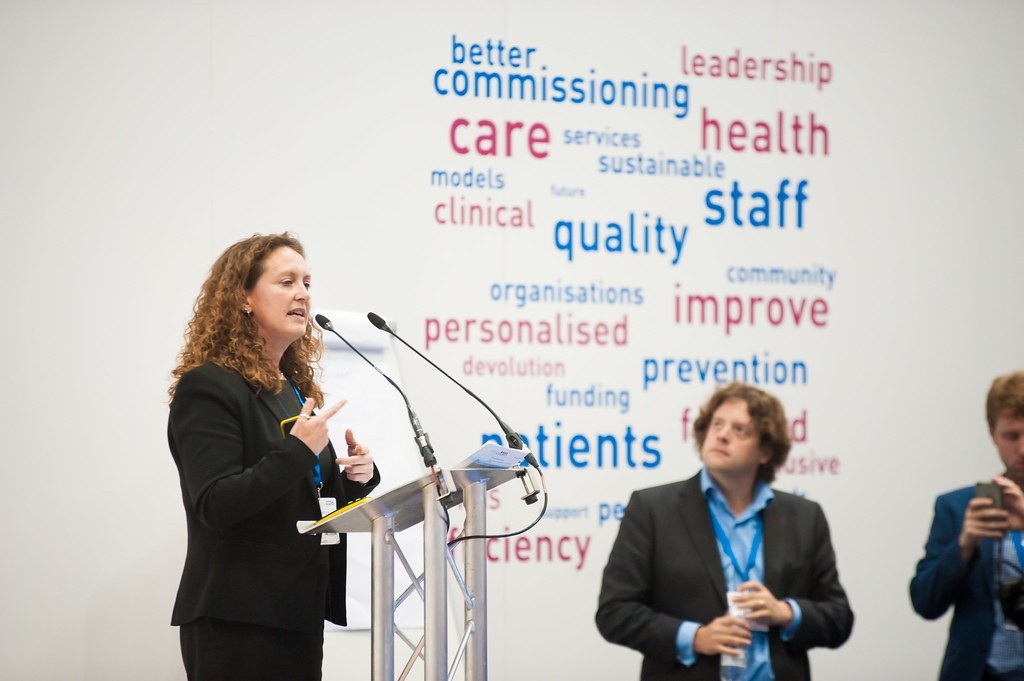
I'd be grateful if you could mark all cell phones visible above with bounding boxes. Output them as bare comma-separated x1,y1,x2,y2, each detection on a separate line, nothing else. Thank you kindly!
974,483,1003,523
280,416,301,441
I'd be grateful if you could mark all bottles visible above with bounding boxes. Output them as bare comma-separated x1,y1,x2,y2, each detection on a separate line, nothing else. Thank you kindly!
719,611,752,681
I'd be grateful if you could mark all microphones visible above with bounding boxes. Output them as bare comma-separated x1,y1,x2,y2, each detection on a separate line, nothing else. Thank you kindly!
366,310,539,468
314,313,436,468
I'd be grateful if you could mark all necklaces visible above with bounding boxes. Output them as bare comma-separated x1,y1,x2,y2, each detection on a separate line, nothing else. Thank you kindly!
268,372,284,380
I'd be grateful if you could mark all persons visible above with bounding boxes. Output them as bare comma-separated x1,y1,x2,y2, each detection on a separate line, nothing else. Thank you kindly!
594,381,854,681
909,368,1024,681
167,231,381,681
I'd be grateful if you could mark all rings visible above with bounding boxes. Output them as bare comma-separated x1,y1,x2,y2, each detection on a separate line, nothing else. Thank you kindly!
299,414,310,420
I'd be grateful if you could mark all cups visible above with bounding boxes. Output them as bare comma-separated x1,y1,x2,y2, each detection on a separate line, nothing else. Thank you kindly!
726,592,753,619
